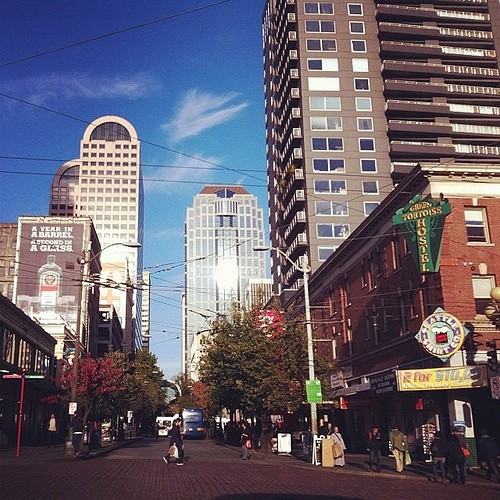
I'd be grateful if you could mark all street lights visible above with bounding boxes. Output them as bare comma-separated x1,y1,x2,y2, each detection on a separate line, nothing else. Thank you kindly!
63,240,142,460
252,244,320,435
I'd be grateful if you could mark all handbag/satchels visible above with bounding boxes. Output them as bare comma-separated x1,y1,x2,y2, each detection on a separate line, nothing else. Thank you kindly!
170,444,179,458
405,451,412,465
332,442,343,458
168,444,175,455
246,440,251,448
461,447,470,456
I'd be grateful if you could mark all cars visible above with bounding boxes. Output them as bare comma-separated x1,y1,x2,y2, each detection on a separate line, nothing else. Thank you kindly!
181,423,208,440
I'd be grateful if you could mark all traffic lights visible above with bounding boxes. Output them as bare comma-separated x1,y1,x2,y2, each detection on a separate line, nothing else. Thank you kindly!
485,339,500,371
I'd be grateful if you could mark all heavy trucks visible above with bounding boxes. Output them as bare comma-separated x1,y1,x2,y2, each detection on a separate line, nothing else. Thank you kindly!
182,406,204,429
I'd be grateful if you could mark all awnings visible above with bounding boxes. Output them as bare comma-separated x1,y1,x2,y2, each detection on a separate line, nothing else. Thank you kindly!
370,364,487,398
329,380,371,399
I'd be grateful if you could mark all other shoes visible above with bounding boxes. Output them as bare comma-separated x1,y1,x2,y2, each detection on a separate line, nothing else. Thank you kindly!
177,463,184,465
163,456,168,464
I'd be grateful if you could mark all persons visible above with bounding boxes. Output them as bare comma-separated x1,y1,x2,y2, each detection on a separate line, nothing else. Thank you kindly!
366,425,384,473
324,422,347,467
163,418,185,466
430,431,467,485
240,421,252,460
391,424,408,473
46,414,56,449
479,428,500,485
